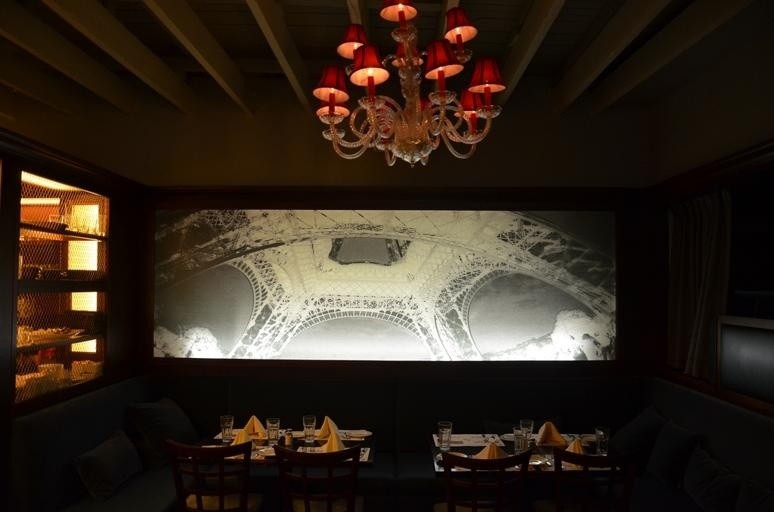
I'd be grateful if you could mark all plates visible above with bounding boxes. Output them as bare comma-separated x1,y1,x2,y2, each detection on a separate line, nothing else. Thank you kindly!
257,447,291,457
281,430,306,438
436,451,468,462
527,455,550,466
345,429,374,438
500,433,516,443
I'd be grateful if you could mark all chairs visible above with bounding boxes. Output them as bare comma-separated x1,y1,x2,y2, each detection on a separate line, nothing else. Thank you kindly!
553,446,623,511
168,440,252,511
442,450,530,512
274,445,360,511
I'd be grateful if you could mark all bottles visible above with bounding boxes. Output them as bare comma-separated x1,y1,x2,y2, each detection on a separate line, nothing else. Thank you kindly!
284,428,294,448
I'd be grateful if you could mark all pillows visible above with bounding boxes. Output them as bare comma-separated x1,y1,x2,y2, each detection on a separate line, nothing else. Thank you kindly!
618,405,774,512
71,429,144,503
127,396,200,469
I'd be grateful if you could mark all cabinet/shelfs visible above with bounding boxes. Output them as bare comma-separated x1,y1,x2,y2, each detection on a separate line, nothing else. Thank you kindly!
16,220,109,397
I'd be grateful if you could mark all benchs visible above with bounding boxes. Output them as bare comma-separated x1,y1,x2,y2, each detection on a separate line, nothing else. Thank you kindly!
227,378,396,512
396,378,585,512
586,376,774,512
6,374,227,511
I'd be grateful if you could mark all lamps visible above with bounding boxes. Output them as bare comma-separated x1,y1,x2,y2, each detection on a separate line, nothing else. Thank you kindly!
310,0,506,169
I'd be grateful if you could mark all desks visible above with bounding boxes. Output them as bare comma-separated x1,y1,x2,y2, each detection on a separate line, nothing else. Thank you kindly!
430,433,617,481
173,426,374,463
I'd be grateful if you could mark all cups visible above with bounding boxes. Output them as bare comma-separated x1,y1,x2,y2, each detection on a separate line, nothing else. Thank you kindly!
512,427,528,456
436,422,453,452
48,209,107,237
13,324,85,396
265,416,281,444
520,419,534,439
302,415,316,443
595,427,609,457
220,415,234,443
17,256,69,280
